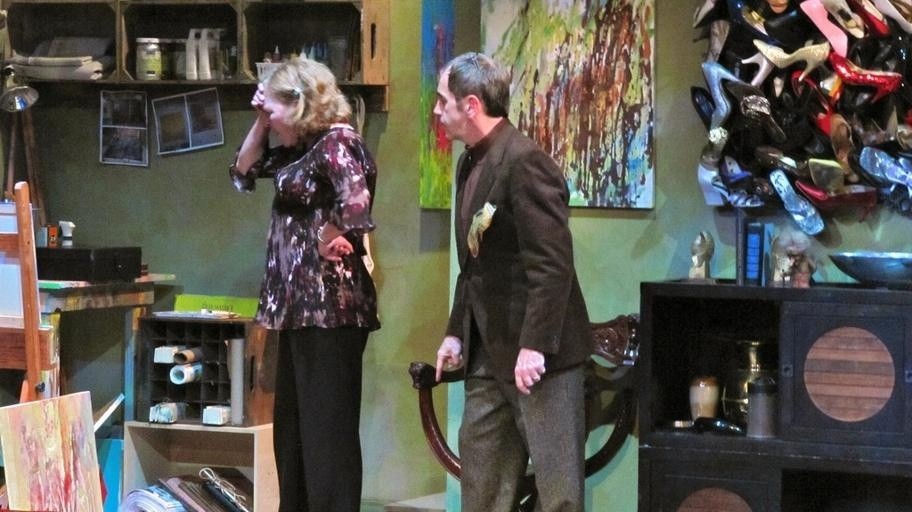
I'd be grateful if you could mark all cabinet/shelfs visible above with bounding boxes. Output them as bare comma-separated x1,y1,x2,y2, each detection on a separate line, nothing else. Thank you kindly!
129,312,277,427
641,277,912,511
1,0,128,89
120,416,283,511
116,2,244,88
235,2,390,114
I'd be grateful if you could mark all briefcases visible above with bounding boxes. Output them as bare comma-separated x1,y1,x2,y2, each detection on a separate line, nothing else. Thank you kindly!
35,245,143,285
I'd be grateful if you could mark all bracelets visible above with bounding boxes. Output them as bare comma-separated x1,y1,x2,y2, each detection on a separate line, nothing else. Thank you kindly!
316,226,332,245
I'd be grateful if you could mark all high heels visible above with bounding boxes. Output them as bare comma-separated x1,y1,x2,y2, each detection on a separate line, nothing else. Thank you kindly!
690,1,911,237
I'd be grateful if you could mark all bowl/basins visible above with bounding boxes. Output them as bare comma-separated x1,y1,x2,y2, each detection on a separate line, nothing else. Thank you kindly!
828,252,911,287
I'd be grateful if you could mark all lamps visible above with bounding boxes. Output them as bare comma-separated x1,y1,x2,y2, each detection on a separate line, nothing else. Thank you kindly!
0,65,42,115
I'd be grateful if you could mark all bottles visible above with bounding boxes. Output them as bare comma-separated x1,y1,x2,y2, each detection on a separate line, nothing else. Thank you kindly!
720,339,778,434
253,42,326,80
689,376,719,425
136,22,231,83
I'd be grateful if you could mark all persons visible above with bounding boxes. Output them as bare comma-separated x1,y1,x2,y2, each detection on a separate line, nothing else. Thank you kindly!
226,53,382,511
430,49,596,512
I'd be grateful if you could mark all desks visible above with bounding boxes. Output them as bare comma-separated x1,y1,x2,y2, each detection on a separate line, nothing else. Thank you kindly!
31,269,178,400
